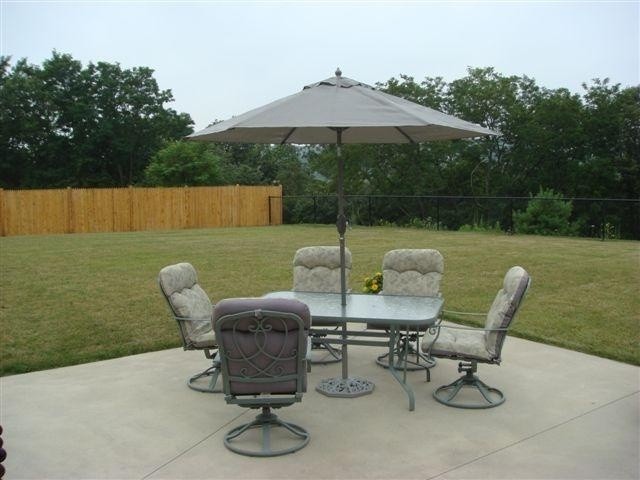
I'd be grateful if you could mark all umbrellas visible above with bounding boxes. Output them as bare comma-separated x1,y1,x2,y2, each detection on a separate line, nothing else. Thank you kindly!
182,65,501,381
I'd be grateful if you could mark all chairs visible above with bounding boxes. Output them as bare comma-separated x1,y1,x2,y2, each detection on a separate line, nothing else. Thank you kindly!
158,262,226,394
210,296,312,456
422,264,531,412
372,249,445,369
291,245,352,364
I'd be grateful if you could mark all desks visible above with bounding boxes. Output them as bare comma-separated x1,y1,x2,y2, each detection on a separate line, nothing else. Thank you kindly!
262,290,441,411
